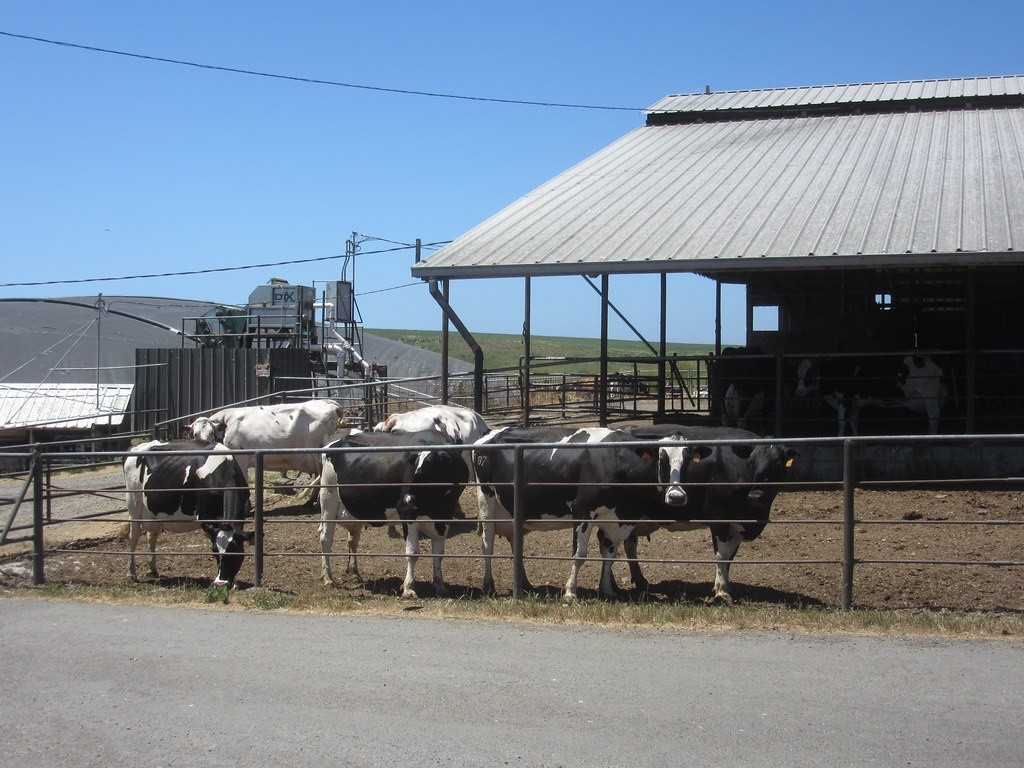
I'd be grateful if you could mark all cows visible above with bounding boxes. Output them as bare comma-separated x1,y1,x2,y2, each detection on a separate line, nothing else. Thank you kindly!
123,437,251,591
349,404,494,484
470,425,713,604
183,398,344,514
316,427,470,600
597,423,800,605
